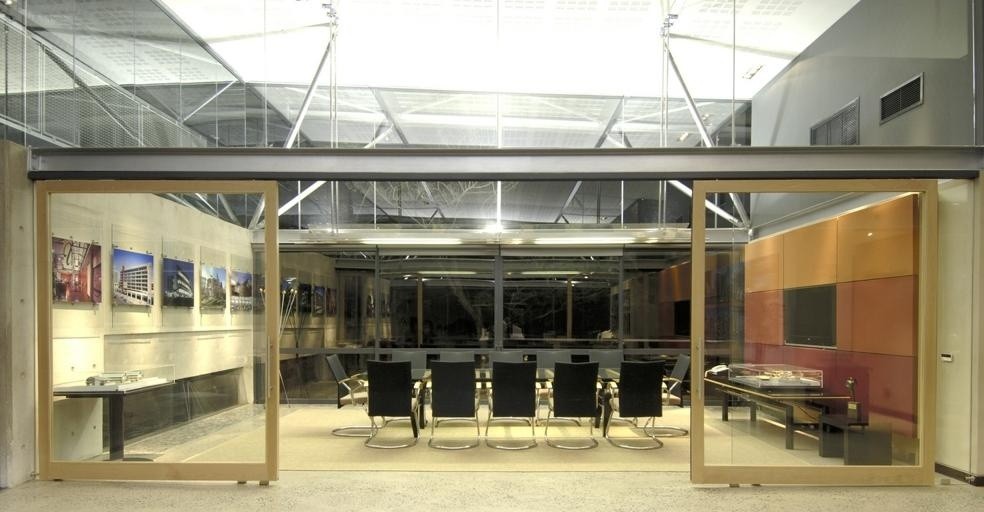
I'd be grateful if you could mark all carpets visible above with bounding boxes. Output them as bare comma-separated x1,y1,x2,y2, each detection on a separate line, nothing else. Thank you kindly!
184,404,815,472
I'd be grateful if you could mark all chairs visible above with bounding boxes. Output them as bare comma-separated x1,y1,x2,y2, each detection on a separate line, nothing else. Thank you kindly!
536,352,581,427
327,354,385,436
389,349,428,428
544,361,601,450
588,351,636,427
605,360,666,450
439,351,477,425
488,350,530,425
635,354,690,437
365,359,421,450
428,360,478,452
484,361,538,450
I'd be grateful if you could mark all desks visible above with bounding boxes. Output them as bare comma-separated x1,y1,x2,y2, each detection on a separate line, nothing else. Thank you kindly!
819,413,893,466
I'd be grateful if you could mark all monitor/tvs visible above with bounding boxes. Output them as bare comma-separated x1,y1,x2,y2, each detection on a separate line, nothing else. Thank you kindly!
782,284,836,346
